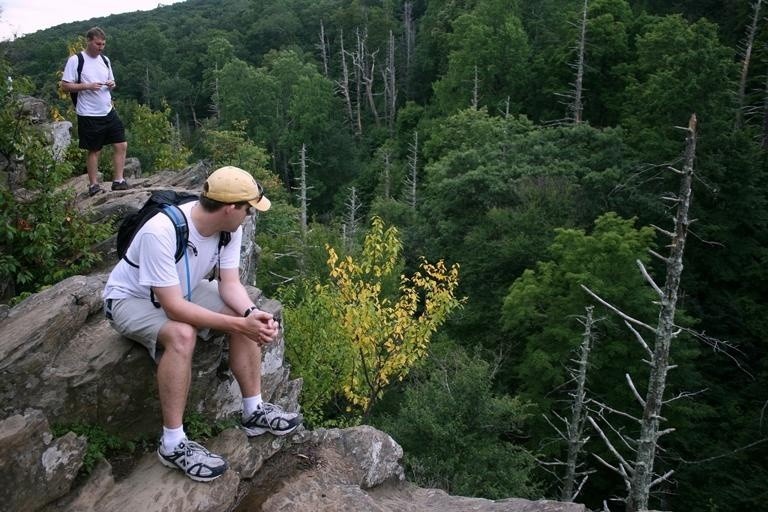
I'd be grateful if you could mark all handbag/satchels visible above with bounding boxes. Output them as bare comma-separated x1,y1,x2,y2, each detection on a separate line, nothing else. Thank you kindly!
244,304,258,320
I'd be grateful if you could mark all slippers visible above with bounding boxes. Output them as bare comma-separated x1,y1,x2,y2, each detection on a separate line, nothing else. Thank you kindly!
117,189,232,268
70,52,109,107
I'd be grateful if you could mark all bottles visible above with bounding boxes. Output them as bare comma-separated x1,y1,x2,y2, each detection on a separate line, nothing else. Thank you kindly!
201,166,272,212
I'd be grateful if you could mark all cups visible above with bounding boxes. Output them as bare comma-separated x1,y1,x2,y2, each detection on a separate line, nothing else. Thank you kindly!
246,183,265,203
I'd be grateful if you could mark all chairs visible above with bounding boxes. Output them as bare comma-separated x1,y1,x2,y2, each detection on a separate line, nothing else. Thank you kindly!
88,182,100,196
156,436,228,482
112,179,129,190
238,403,300,437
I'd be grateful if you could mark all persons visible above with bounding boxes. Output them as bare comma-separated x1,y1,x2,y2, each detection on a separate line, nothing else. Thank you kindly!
59,25,132,198
103,164,305,485
